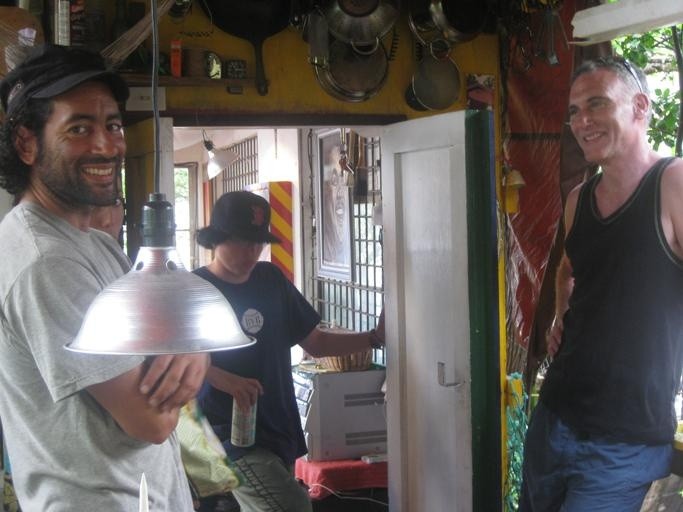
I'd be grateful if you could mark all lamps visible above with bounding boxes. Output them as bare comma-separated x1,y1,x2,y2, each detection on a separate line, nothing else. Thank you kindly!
193,109,240,184
58,0,257,361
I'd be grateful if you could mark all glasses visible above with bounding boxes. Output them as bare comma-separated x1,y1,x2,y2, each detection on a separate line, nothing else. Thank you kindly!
592,54,644,92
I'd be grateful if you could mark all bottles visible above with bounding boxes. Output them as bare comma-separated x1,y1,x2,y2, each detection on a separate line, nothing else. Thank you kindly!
3,27,38,75
230,394,257,448
111,1,148,70
45,0,72,45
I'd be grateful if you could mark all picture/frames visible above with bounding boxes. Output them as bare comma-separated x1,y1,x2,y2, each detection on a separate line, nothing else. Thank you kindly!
308,122,358,288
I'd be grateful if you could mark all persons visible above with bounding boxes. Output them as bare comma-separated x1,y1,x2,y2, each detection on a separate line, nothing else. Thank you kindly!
189,190,385,512
89,168,124,241
516,57,683,512
0,45,212,512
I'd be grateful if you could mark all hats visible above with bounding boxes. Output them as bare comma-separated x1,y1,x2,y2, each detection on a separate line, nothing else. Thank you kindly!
211,190,281,243
1,42,129,121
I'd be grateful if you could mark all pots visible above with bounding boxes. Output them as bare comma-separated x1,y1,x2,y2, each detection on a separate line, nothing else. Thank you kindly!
312,39,392,103
322,0,400,56
407,0,491,62
195,0,295,97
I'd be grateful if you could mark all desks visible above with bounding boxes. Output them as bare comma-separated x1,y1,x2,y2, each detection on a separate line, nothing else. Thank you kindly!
289,451,388,511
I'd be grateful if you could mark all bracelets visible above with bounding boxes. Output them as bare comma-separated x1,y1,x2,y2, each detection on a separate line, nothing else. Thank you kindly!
369,329,386,350
